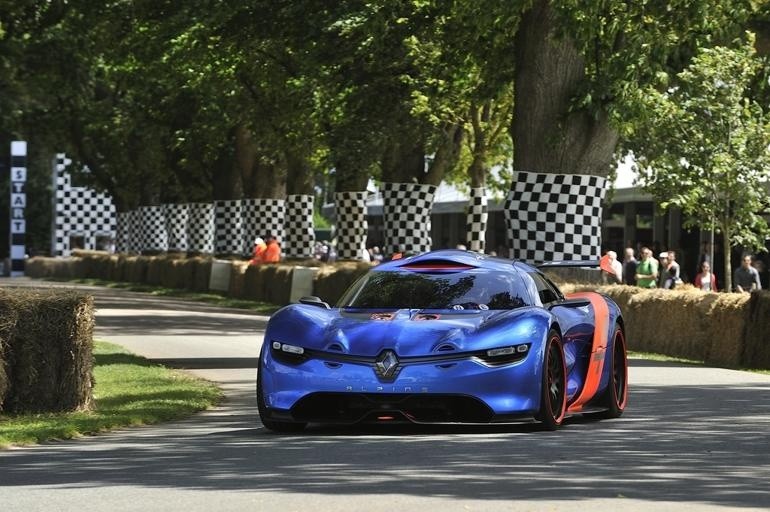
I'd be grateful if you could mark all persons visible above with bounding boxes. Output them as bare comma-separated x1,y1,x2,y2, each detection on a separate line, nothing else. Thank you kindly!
368,246,385,261
248,234,281,265
734,252,770,293
602,245,680,290
695,261,718,292
314,240,337,263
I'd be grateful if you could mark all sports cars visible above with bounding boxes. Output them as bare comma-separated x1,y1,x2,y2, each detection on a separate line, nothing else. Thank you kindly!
256,248,629,432
